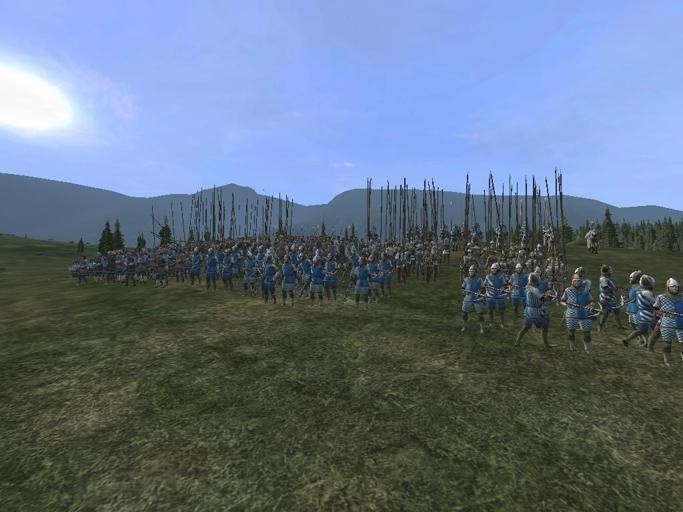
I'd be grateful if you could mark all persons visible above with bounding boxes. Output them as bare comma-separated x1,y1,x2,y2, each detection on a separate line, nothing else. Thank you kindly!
68,221,682,369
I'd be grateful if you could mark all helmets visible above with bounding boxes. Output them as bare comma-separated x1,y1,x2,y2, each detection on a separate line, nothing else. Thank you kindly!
528,272,541,286
468,264,522,269
629,271,680,291
601,264,612,275
571,267,586,285
